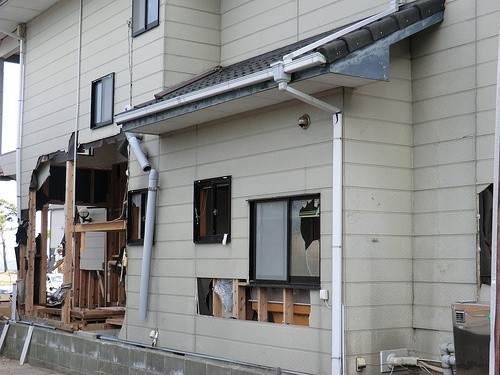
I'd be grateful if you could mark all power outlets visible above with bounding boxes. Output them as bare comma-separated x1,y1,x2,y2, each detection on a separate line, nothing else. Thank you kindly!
380,348,409,373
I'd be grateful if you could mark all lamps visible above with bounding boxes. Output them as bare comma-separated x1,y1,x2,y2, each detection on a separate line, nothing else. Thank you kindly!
77,207,92,223
